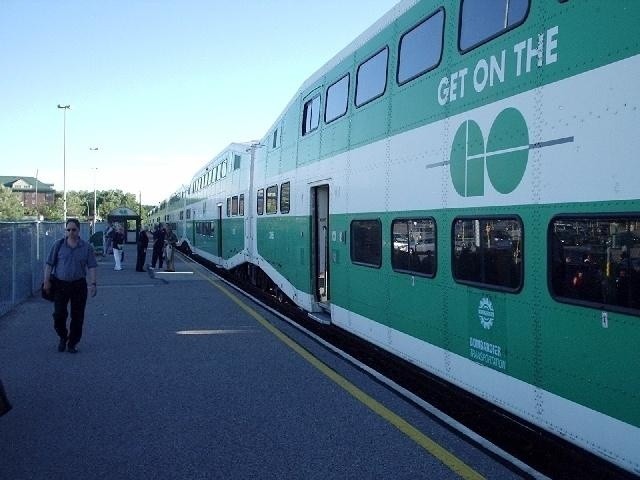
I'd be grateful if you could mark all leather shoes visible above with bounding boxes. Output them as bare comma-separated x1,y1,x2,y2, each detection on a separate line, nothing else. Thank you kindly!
59,333,69,351
68,342,78,352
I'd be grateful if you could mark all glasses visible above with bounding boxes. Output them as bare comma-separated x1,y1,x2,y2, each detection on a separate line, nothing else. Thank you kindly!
67,229,76,232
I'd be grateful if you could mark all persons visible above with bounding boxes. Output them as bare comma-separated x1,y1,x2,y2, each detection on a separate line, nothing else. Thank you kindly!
103,223,179,273
394,219,640,306
43,219,98,353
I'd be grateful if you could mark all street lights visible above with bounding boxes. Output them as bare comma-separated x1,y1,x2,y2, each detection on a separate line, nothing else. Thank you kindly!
89,147,100,230
57,104,71,229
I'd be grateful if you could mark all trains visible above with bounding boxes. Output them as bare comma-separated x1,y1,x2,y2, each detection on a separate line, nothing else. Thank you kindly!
146,0,639,475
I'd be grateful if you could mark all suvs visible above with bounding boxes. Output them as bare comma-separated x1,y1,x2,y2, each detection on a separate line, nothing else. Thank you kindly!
409,237,435,255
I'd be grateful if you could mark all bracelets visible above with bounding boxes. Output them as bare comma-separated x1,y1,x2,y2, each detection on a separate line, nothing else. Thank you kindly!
90,280,97,287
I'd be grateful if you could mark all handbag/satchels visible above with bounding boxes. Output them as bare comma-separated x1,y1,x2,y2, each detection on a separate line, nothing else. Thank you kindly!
42,274,56,301
